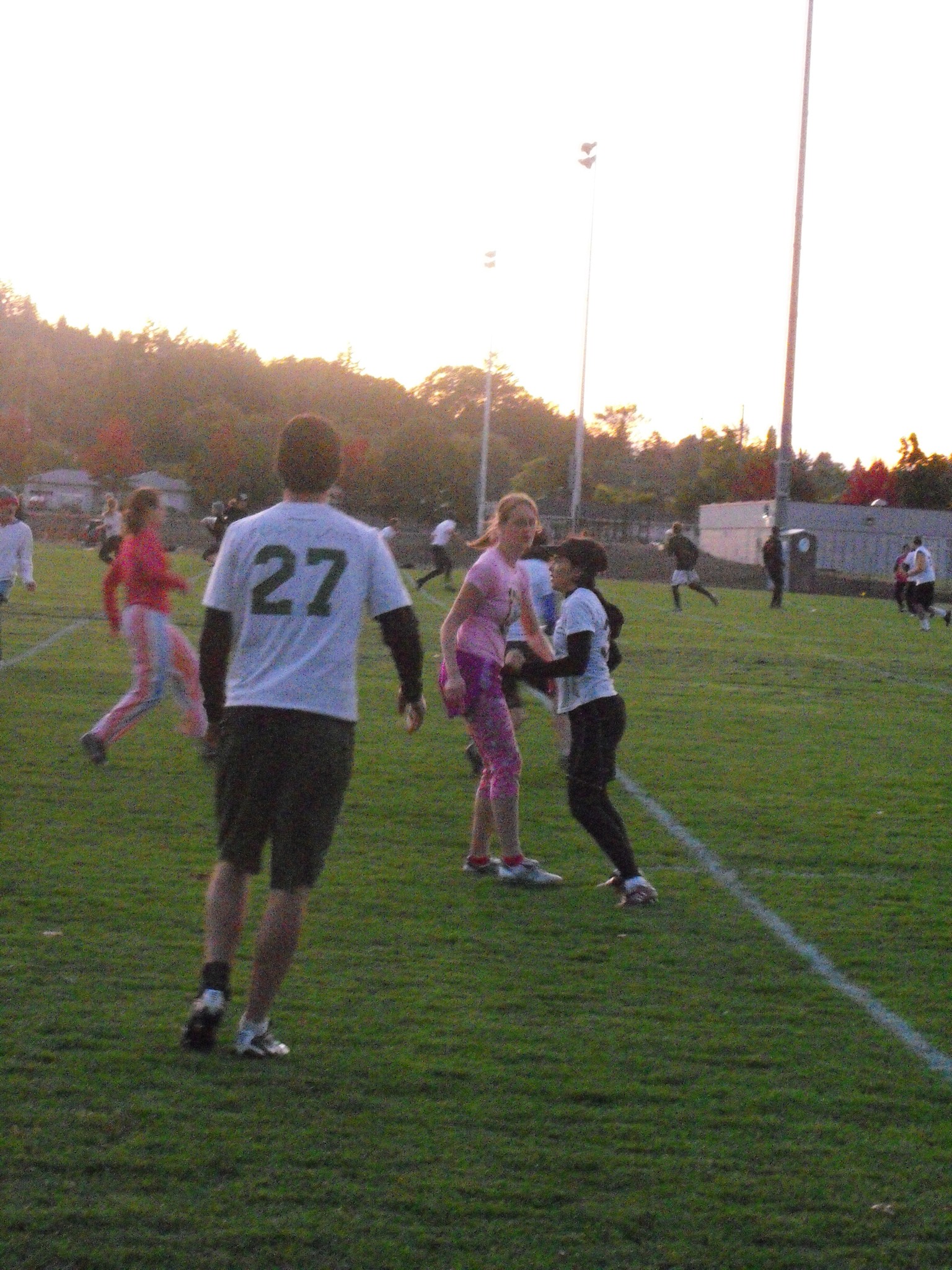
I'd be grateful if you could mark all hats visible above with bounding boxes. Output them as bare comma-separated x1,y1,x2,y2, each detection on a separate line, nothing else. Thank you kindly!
539,536,608,574
237,494,248,501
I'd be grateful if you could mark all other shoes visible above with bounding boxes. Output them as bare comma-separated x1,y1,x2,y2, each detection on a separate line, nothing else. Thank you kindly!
414,579,423,590
199,745,219,762
558,754,568,768
80,733,105,765
465,743,483,773
444,586,457,594
944,610,951,628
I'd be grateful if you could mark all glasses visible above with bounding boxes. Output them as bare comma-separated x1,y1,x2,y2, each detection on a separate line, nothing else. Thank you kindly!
505,518,537,529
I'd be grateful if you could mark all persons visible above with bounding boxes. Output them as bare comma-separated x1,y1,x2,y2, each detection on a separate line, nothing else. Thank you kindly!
415,511,457,593
506,536,657,907
761,526,786,608
893,536,951,632
0,486,36,603
466,527,557,775
201,490,275,567
86,491,123,565
438,494,562,882
664,523,720,613
77,486,213,768
182,413,426,1059
15,492,44,520
378,518,401,553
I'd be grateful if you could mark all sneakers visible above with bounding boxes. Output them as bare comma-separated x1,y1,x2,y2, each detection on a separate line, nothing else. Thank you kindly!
234,1031,290,1059
615,883,658,910
498,858,563,886
464,858,500,877
180,999,223,1053
596,875,622,890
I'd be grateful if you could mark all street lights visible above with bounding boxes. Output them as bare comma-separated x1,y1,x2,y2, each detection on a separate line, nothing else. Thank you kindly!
475,250,498,538
568,139,597,526
